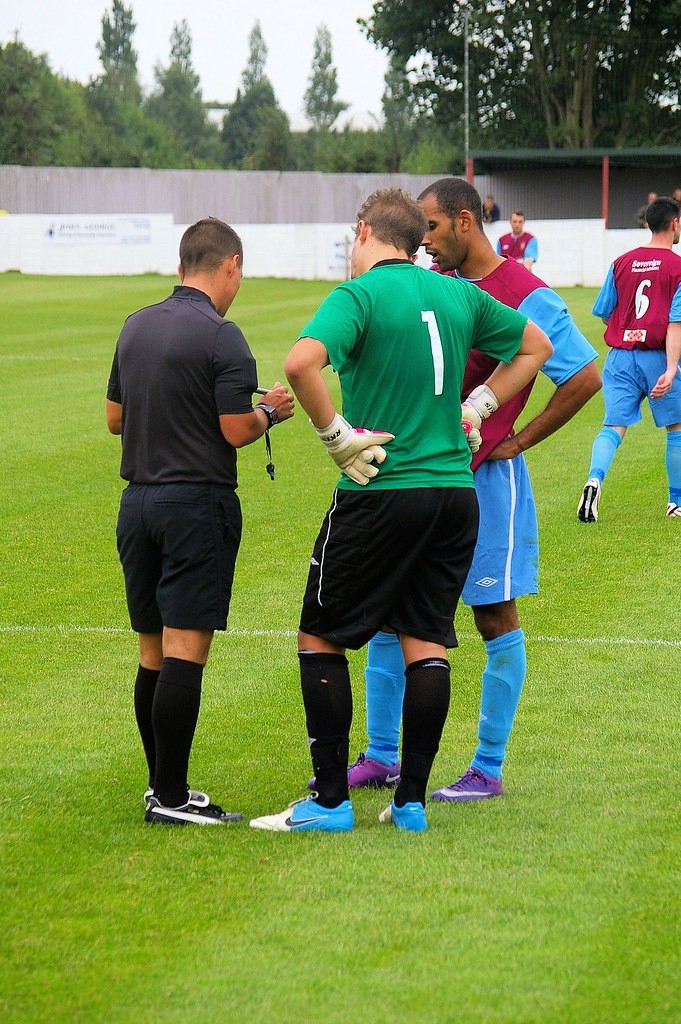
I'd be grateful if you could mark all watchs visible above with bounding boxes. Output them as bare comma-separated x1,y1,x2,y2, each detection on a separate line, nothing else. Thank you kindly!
255,403,278,429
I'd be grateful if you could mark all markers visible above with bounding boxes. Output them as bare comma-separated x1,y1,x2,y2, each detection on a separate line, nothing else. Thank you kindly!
254,388,270,395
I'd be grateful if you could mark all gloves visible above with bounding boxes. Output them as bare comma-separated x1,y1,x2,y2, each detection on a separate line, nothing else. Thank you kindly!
460,384,499,453
316,415,396,487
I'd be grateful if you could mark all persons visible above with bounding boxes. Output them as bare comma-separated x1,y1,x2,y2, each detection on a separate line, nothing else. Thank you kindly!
306,179,603,801
673,189,681,203
636,192,657,228
106,217,295,824
495,210,538,273
577,196,681,523
249,187,556,832
481,195,500,223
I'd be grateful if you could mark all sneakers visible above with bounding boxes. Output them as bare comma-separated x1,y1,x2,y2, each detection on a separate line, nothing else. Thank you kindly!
378,799,427,833
144,790,154,804
665,502,681,517
431,767,503,802
576,477,601,522
144,796,243,827
249,791,354,832
307,753,401,789
